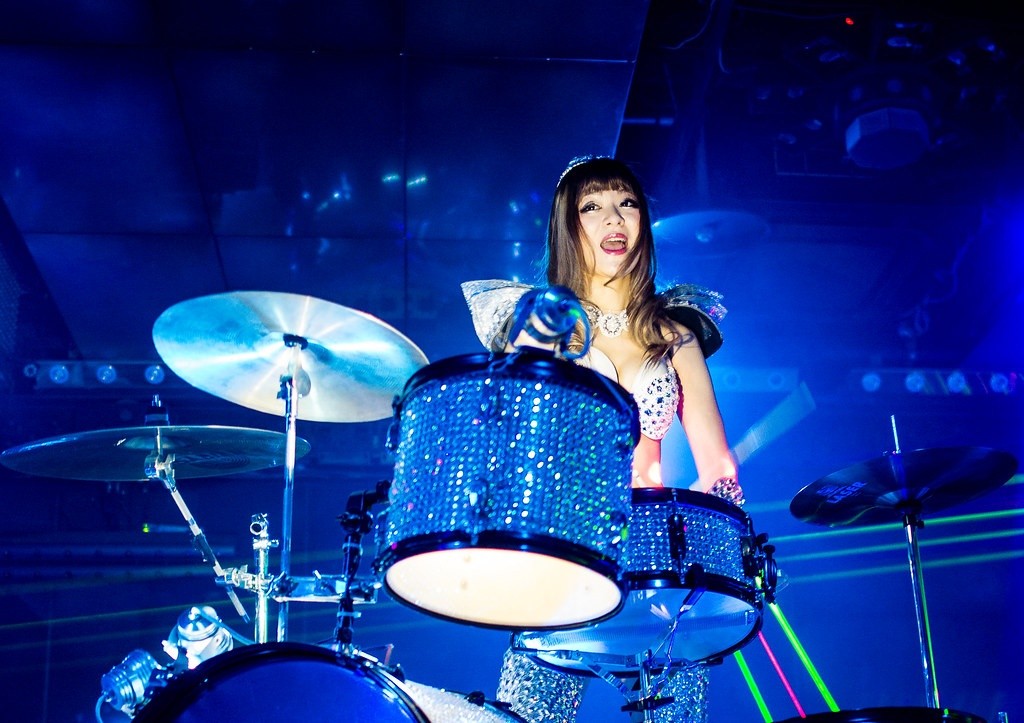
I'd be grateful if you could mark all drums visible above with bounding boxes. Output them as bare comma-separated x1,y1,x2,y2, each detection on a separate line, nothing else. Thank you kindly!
378,347,642,632
132,641,532,723
512,486,768,677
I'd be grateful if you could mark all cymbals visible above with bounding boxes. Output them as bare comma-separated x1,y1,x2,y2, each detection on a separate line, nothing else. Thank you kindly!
788,444,1018,528
0,424,311,482
151,291,431,425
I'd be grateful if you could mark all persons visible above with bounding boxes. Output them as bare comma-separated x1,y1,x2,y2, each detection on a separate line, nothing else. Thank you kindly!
495,158,745,723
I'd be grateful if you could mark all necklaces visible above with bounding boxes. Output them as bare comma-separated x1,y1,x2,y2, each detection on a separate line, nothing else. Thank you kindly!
580,300,629,336
